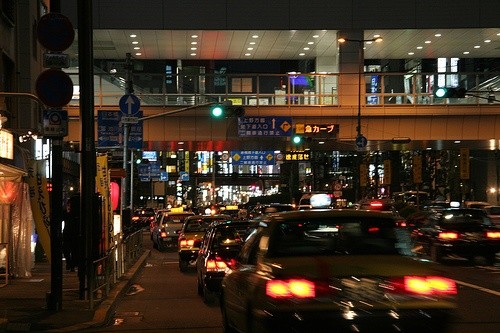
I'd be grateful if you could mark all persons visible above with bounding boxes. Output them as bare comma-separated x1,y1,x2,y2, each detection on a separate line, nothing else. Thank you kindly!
487,86,494,103
62,238,76,272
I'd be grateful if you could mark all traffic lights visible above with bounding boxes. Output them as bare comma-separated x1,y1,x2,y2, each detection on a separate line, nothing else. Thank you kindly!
292,136,311,144
433,86,466,99
211,105,245,121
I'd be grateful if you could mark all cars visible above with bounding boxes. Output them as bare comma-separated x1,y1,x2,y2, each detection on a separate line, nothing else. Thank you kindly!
150,194,500,333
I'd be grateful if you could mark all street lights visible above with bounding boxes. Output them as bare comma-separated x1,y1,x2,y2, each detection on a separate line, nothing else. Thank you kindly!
337,37,383,133
120,100,224,236
